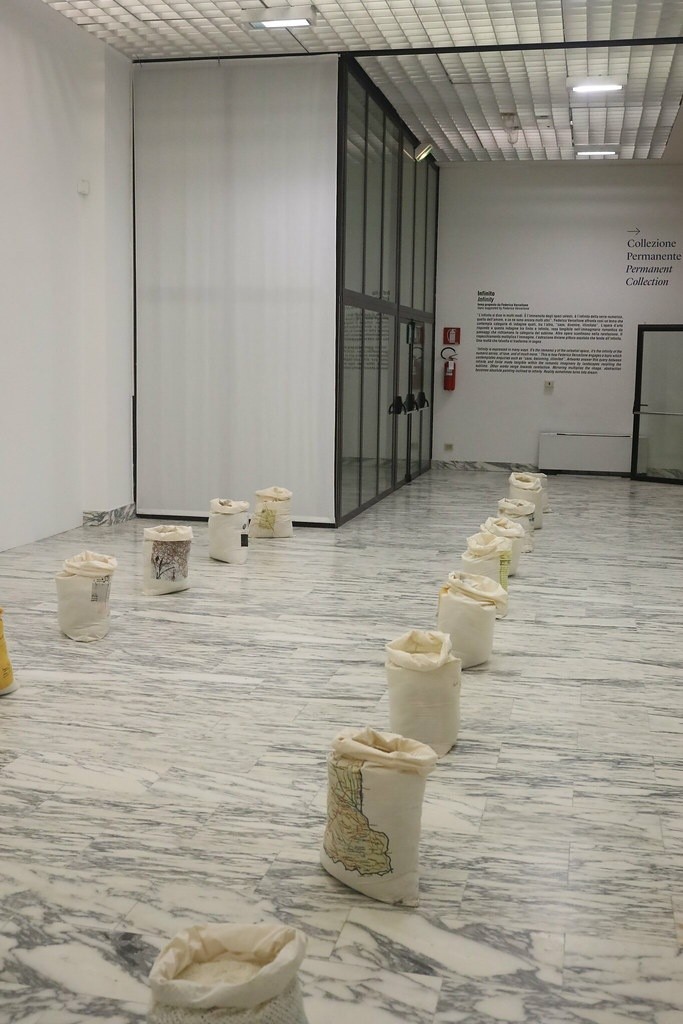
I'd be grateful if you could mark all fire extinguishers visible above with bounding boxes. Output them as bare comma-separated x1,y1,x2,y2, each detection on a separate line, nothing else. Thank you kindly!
441,347,457,390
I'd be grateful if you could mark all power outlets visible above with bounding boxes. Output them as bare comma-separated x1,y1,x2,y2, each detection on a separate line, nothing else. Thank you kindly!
444,444,453,450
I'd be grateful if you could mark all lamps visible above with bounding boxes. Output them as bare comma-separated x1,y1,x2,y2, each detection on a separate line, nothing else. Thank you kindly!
241,4,317,31
575,145,621,156
414,143,433,162
566,74,628,92
